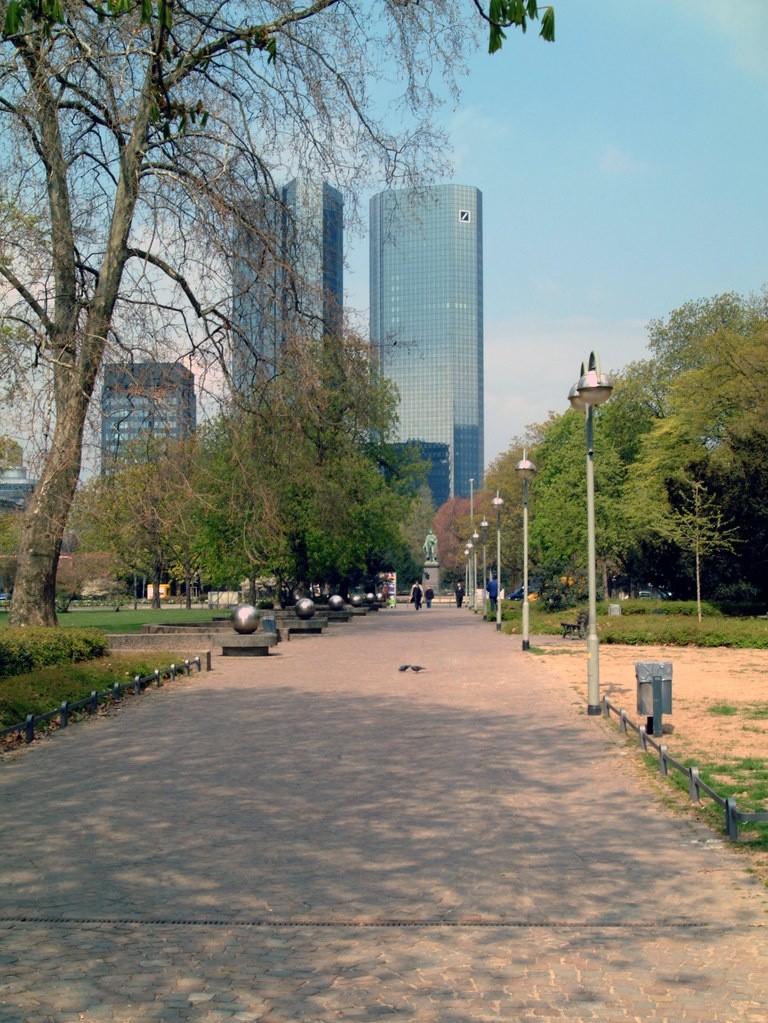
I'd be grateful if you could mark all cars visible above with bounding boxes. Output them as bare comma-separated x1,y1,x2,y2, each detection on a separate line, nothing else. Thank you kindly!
505,585,540,602
626,589,671,601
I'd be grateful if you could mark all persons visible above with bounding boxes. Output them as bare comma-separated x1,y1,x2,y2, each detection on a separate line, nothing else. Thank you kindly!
486,575,503,612
423,530,437,562
408,581,424,610
455,582,465,608
384,582,389,591
389,594,394,608
387,572,395,580
424,585,434,608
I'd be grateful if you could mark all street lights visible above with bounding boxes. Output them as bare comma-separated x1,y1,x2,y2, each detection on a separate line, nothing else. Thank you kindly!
490,490,504,630
479,516,489,620
567,350,615,715
515,448,538,650
464,528,479,613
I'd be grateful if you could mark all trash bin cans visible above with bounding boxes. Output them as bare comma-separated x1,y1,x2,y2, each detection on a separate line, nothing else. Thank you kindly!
636,660,674,715
608,604,621,616
262,616,276,633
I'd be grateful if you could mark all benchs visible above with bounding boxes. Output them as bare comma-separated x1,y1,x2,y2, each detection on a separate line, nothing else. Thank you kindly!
561,612,589,640
